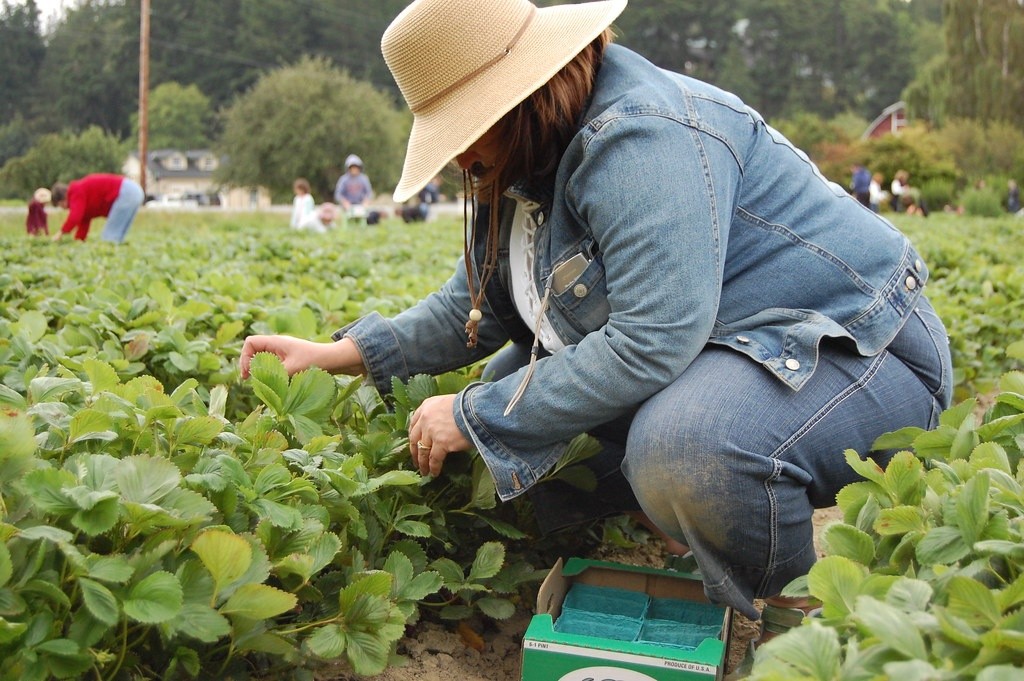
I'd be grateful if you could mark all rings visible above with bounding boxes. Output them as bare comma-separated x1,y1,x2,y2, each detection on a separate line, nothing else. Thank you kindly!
417,439,431,449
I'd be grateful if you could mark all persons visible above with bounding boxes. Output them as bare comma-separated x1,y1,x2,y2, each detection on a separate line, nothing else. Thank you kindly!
52,173,145,243
943,178,1024,214
237,0,953,681
26,187,52,237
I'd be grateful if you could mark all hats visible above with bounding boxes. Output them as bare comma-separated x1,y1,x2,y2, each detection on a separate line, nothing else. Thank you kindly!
380,0,628,203
35,188,51,203
345,155,363,167
318,203,336,219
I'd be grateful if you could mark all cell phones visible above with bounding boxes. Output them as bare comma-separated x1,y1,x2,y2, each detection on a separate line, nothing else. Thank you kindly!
550,253,592,296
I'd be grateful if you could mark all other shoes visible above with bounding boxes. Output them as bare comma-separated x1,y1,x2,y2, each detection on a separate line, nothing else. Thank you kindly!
664,550,701,576
745,604,822,660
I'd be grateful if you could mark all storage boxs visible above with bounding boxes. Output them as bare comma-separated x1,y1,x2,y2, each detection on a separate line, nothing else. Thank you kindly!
518,556,732,681
